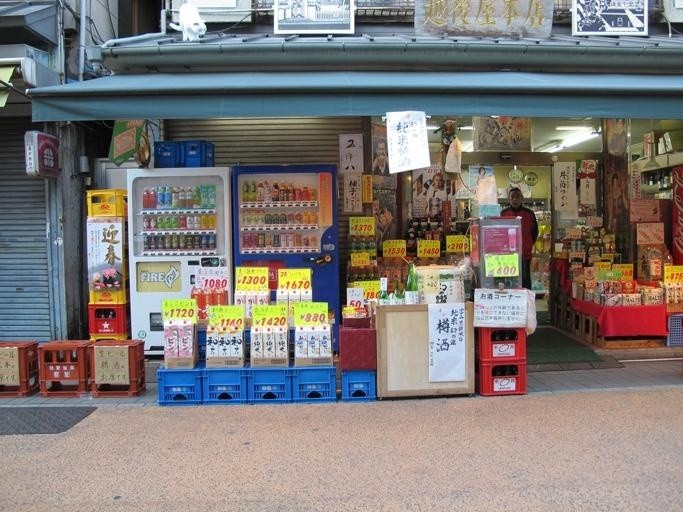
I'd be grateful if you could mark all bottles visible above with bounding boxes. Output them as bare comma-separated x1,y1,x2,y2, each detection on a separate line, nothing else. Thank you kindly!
379,260,420,305
142,183,217,250
240,178,320,249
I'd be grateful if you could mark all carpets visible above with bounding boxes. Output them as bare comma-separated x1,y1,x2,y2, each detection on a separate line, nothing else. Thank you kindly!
525,311,606,364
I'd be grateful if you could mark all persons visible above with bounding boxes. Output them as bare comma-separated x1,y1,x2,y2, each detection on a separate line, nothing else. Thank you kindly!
372,118,630,251
500,188,538,288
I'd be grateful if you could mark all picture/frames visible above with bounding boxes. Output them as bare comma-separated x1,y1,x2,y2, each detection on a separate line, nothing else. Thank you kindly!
572,0,649,36
273,0,354,35
